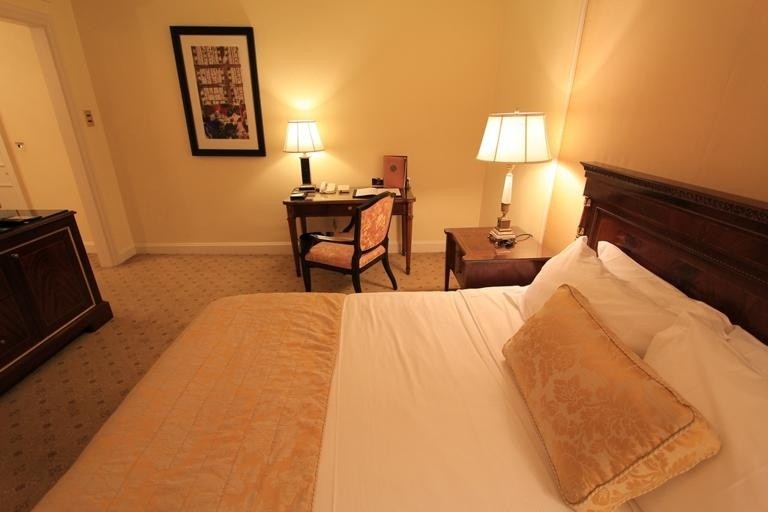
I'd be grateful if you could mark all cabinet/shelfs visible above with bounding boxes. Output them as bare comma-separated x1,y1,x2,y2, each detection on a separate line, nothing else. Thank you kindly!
0,208,114,398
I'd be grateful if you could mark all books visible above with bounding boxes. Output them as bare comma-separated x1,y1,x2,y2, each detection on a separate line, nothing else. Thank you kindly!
384,156,407,187
353,188,401,197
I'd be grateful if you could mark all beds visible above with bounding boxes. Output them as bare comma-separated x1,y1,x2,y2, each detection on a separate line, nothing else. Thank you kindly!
31,162,767,512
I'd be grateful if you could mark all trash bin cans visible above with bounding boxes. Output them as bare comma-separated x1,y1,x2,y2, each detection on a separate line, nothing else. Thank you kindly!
300,232,324,267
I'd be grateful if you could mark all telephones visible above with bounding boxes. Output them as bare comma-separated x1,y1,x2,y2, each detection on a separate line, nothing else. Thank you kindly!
319,182,336,194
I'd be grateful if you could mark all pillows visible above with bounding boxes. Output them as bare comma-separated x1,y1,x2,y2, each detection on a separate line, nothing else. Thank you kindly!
633,315,767,512
510,285,725,511
596,241,728,335
512,236,677,360
726,326,765,380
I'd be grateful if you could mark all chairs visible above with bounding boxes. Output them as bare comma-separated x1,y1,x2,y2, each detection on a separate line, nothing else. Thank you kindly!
302,191,397,292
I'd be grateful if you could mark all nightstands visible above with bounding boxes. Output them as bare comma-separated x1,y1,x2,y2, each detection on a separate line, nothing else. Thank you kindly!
444,227,551,289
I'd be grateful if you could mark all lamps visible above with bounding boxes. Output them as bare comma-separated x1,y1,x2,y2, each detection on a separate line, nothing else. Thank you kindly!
474,112,553,246
283,121,324,184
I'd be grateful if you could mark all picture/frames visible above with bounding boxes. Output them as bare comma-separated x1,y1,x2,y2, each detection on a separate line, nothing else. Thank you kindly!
170,26,267,157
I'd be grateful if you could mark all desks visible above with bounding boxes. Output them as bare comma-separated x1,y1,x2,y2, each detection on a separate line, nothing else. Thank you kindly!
283,186,416,277
